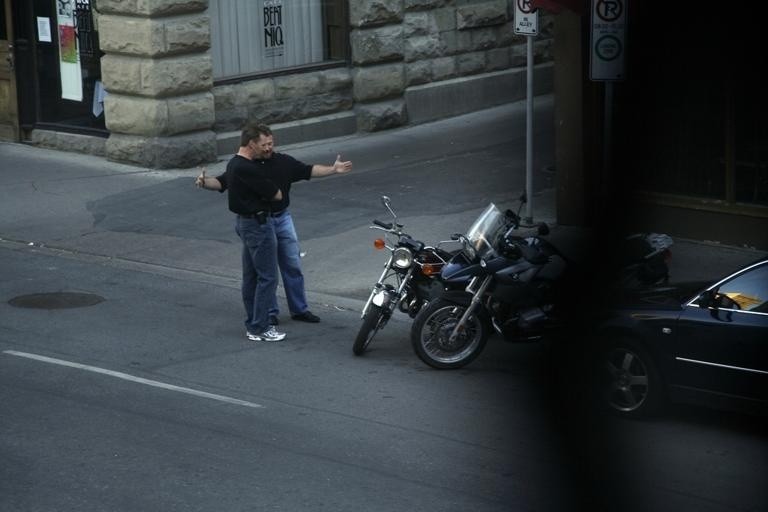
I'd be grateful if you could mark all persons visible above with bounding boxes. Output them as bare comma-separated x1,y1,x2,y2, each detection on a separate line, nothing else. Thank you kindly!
222,124,290,344
192,124,355,326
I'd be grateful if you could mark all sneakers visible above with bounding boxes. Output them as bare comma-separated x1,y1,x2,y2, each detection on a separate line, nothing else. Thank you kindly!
246,325,287,341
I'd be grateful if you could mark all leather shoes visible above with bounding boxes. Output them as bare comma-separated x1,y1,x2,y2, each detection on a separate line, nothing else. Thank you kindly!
267,315,279,325
291,311,321,323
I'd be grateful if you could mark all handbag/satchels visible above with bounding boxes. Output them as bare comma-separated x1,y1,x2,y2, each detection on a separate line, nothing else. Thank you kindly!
256,211,267,225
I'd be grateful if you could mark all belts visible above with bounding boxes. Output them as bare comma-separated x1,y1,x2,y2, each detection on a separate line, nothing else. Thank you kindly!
237,214,256,219
272,210,284,218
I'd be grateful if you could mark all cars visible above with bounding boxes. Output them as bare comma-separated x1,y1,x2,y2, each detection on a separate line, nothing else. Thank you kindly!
609,256,768,418
